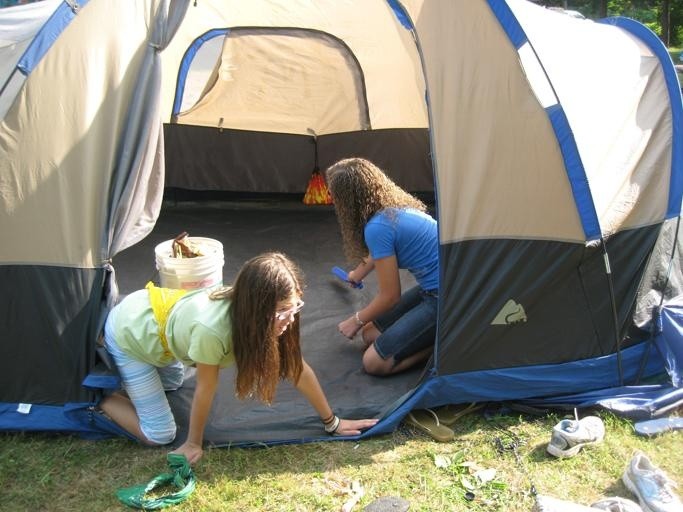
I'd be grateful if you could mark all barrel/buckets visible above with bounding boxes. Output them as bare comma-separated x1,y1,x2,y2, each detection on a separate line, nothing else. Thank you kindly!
153,236,224,294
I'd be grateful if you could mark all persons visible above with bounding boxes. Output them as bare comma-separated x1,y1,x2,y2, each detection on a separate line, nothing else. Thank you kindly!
326,157,440,377
99,252,380,470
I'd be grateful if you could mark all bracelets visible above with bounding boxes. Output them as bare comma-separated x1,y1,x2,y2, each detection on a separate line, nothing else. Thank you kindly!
356,312,368,327
321,414,340,433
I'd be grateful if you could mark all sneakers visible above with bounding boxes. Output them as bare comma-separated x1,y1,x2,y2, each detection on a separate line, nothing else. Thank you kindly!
621,452,682,512
546,415,604,457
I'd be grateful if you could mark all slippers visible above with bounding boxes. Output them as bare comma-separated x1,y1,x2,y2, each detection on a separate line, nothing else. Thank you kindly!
433,399,488,424
403,408,454,443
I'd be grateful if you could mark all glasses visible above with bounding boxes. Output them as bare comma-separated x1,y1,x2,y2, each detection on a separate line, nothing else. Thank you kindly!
271,299,306,321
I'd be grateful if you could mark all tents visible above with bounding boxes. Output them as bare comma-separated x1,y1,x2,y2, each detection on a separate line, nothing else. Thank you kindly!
0,0,683,444
